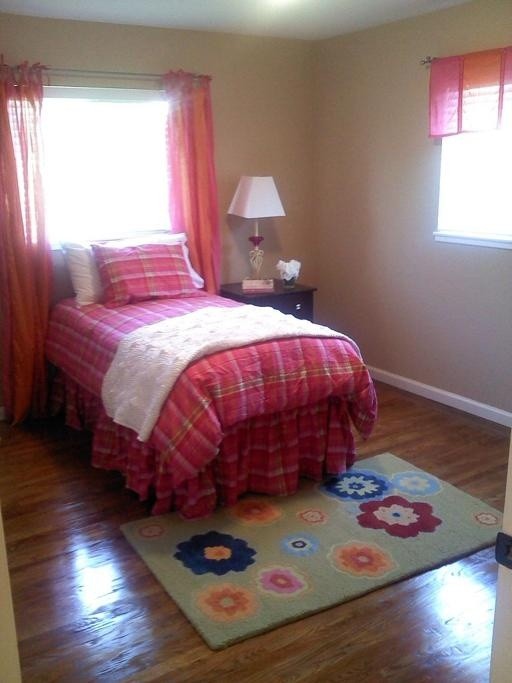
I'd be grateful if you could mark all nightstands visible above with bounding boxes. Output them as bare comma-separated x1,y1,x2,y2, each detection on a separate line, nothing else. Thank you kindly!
219,279,317,326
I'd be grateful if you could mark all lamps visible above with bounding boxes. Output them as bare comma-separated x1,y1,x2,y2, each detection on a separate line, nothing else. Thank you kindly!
225,173,288,291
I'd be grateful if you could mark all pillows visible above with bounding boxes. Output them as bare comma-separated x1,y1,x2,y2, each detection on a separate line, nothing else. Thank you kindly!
60,233,204,306
92,241,198,308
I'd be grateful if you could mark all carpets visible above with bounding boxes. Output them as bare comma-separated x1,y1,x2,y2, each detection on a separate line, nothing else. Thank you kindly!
119,445,507,650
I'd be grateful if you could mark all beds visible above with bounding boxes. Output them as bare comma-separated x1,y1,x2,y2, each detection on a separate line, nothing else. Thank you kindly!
39,288,381,506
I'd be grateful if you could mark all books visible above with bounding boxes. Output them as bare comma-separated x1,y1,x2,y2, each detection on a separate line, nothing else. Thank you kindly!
242,279,274,293
